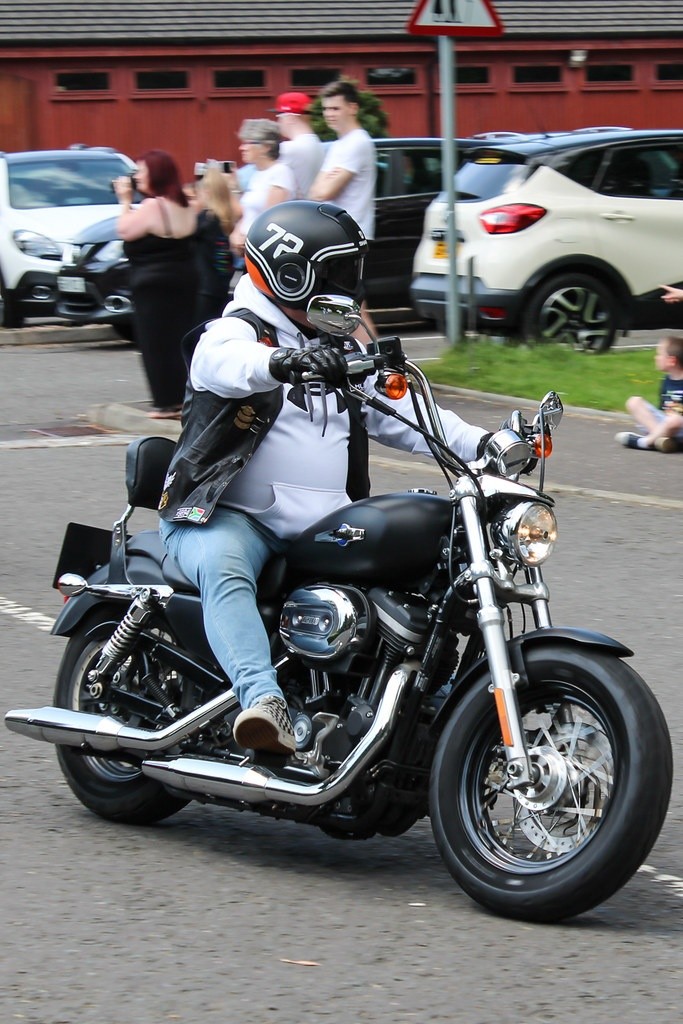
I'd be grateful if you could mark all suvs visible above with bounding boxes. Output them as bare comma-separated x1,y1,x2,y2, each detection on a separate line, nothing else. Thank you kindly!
0,144,148,323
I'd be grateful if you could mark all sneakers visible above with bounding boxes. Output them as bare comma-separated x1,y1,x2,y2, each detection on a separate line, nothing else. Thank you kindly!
233,695,296,755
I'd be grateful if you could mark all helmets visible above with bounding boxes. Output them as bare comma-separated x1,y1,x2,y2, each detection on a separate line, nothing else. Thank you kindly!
244,201,369,331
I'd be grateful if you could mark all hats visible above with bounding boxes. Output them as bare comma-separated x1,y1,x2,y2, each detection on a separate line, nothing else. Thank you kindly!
264,92,313,114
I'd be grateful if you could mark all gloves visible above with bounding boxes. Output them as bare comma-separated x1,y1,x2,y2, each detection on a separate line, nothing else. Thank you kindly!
269,347,348,385
477,419,538,474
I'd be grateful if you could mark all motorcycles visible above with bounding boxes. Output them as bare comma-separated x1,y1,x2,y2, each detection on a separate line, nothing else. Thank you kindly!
0,295,675,927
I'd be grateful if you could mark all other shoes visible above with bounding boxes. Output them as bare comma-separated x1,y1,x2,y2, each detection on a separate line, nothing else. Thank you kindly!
614,431,649,450
149,405,184,419
654,437,677,451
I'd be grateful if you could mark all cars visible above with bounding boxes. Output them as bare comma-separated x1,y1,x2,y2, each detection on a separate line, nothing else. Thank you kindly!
57,136,495,339
401,132,683,356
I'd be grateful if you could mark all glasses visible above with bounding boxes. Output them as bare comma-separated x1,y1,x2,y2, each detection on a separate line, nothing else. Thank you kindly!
322,107,342,111
239,141,267,146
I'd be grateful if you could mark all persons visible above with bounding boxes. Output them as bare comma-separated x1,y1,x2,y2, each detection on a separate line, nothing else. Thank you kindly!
158,202,538,757
112,82,377,418
615,283,683,453
